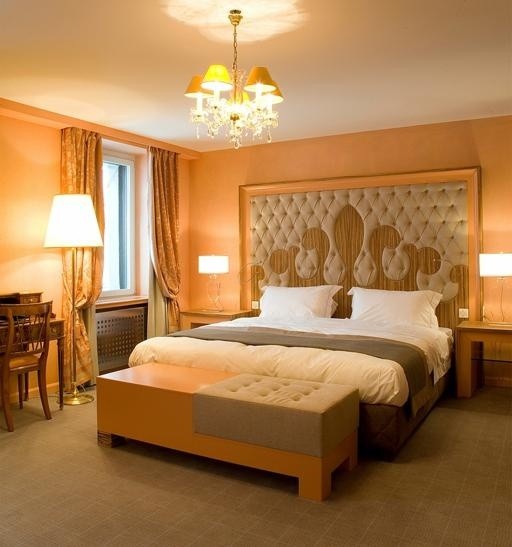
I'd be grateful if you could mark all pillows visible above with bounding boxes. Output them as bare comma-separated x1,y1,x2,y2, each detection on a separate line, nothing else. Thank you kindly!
258,284,343,319
347,286,442,328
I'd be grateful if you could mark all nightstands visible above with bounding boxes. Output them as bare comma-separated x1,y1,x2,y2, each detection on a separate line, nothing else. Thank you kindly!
454,319,511,399
178,308,254,330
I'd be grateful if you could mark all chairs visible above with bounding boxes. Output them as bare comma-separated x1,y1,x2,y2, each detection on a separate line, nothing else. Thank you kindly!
1,299,55,431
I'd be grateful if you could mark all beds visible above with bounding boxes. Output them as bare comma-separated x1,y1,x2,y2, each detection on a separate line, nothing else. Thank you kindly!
128,315,453,461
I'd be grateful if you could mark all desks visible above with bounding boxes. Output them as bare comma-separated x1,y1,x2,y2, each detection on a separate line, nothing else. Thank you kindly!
1,291,68,410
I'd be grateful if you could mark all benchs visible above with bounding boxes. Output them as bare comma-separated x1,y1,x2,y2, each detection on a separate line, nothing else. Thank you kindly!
96,360,360,500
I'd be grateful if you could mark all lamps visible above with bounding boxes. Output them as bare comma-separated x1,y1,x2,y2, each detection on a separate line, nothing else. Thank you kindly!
199,254,230,312
477,250,511,326
184,22,285,150
43,193,104,404
